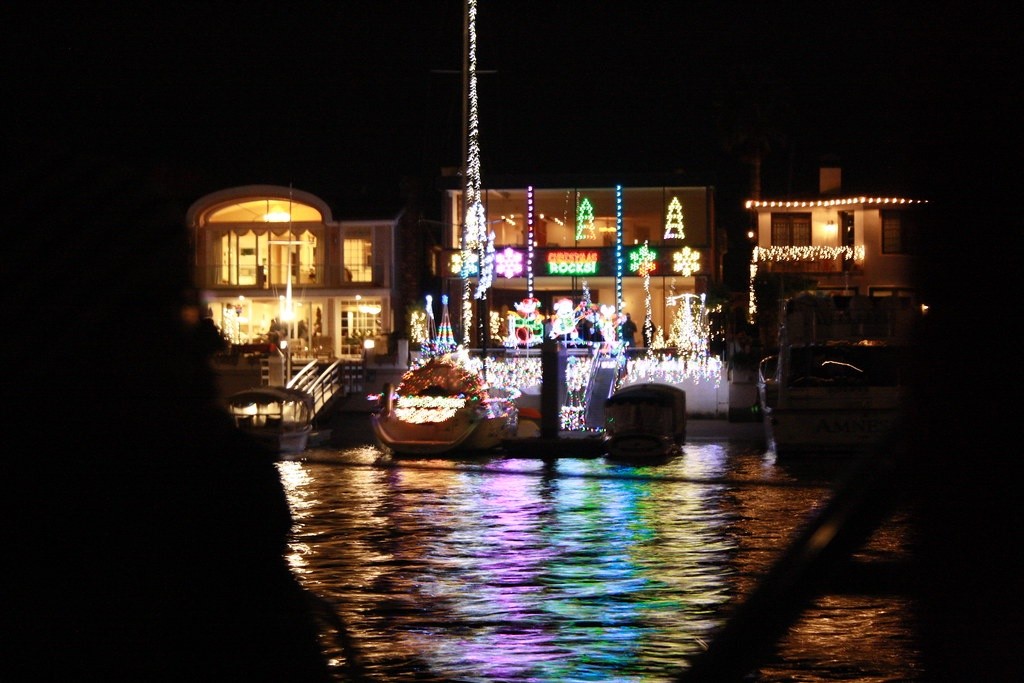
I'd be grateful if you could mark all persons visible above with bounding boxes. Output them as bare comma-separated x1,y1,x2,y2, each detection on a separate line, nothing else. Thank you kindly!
579,313,658,349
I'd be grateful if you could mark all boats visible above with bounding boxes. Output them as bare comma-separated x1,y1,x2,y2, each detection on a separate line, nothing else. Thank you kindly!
372,361,553,462
756,296,914,461
227,389,314,455
603,383,688,465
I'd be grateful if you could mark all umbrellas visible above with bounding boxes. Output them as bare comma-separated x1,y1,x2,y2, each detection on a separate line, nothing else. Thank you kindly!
437,296,453,341
425,296,435,342
